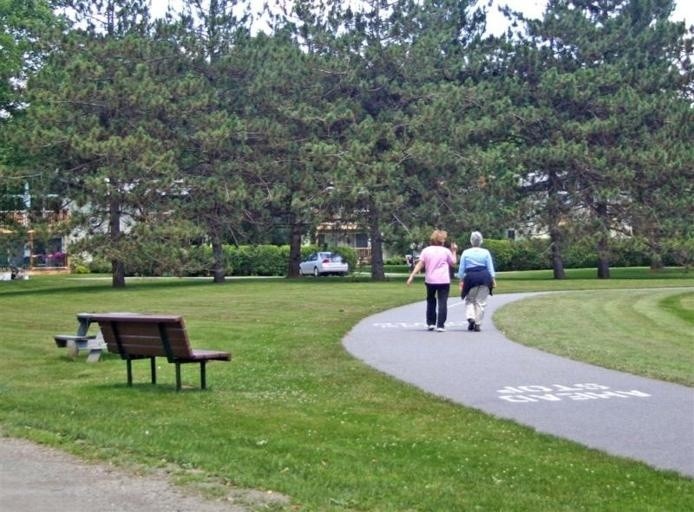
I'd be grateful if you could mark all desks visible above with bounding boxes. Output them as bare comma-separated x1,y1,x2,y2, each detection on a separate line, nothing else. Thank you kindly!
66,314,107,362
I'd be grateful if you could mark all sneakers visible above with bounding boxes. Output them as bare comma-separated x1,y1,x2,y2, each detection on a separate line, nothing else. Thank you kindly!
467,317,481,333
426,324,447,332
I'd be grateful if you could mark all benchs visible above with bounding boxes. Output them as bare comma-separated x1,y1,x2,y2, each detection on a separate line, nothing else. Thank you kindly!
87,314,230,392
53,336,95,349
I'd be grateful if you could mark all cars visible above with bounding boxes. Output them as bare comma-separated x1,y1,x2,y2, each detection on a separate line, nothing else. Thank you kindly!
297,250,349,277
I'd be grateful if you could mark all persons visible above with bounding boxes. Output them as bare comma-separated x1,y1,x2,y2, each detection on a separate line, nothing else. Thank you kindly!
458,231,497,332
407,255,415,269
407,229,457,331
